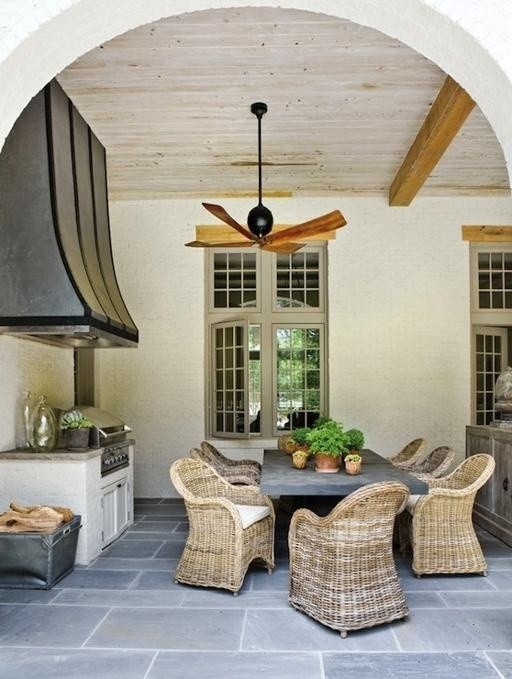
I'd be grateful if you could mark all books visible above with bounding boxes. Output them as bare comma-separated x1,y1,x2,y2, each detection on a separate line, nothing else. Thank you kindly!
0,515,82,591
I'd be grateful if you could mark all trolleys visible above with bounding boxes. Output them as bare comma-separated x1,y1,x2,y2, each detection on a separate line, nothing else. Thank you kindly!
186,102,346,258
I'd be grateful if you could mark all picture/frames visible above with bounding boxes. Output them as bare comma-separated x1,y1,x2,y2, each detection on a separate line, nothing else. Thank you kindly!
258,447,429,497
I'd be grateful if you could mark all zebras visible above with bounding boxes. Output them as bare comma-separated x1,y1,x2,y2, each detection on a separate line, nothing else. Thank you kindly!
67,405,136,478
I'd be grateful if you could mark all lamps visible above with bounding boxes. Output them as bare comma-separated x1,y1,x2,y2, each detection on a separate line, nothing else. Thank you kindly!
286,481,412,636
169,440,276,597
389,437,496,578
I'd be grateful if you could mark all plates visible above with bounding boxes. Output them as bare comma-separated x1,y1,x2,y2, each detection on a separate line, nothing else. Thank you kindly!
462,425,510,544
101,476,132,551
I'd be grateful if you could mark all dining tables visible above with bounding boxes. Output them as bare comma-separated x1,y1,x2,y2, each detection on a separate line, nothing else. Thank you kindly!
277,420,366,474
59,407,95,452
